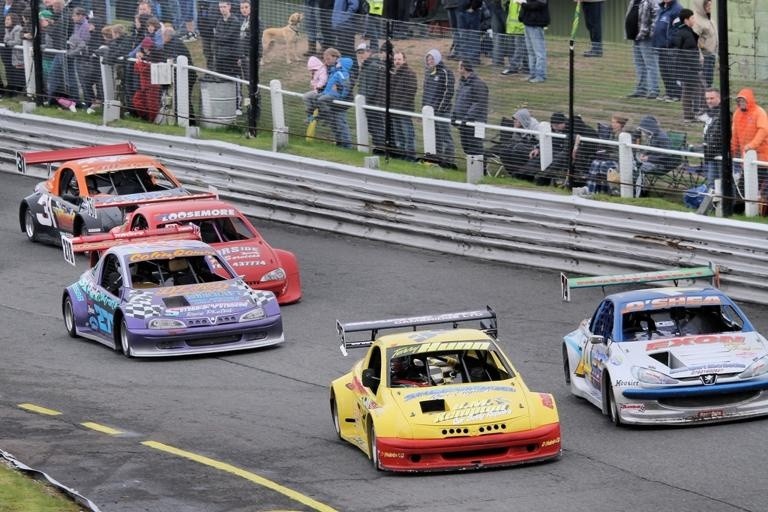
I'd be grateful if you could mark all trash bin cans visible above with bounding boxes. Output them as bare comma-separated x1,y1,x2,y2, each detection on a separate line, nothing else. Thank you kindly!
198,75,237,130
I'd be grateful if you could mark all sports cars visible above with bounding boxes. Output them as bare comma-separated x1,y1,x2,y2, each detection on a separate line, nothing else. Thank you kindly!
16,140,192,246
85,183,307,309
560,258,768,431
325,302,563,476
60,223,292,361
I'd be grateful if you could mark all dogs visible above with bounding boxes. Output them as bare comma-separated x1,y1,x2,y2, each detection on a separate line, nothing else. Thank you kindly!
260,12,306,65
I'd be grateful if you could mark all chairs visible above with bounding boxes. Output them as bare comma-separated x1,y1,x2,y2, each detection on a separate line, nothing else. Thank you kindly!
474,116,709,194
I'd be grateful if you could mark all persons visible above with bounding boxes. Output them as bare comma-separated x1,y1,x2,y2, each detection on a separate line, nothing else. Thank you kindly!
730,88,768,203
67,176,93,205
302,1,721,205
0,1,262,138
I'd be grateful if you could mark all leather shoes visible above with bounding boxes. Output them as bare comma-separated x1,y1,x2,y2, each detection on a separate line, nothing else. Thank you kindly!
627,91,679,102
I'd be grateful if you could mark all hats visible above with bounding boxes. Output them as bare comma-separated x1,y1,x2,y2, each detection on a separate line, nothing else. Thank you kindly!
140,38,152,47
38,9,52,19
354,43,369,53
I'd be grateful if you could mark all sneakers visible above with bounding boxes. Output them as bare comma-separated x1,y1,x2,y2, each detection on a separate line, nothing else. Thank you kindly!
694,111,708,122
529,77,543,82
